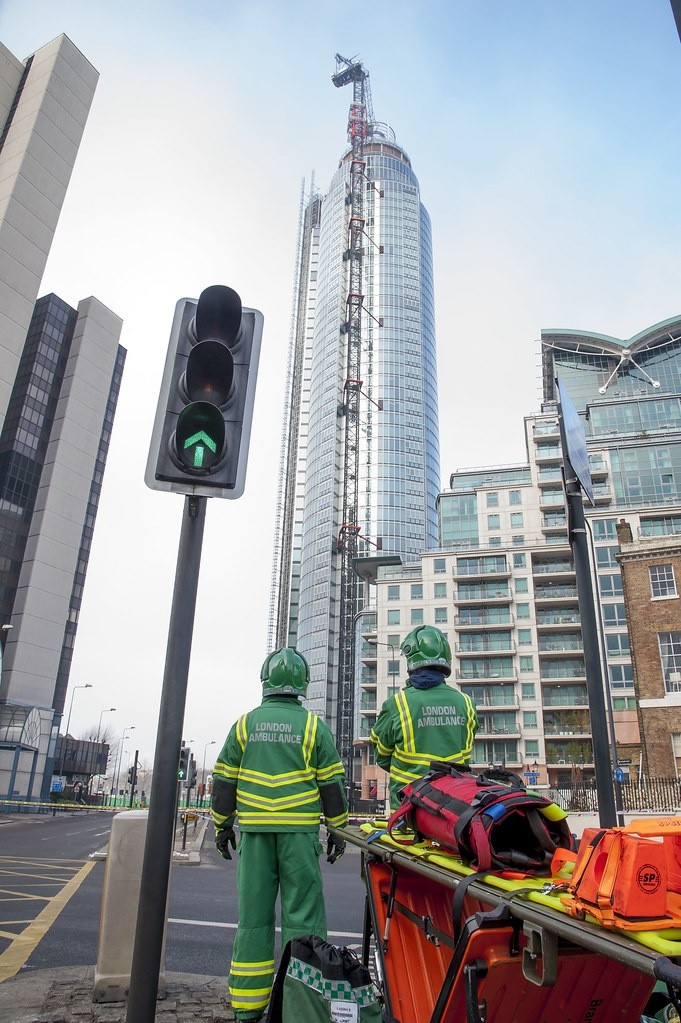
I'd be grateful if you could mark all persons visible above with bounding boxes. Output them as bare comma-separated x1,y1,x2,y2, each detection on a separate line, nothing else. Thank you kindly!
208,648,350,1023
370,624,479,819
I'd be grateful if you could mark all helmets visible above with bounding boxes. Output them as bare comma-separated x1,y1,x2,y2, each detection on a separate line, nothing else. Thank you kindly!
261,647,311,698
400,625,452,678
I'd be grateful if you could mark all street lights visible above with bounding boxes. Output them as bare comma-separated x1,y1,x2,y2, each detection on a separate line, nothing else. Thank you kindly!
109,736,131,810
52,684,92,815
199,741,216,813
87,708,117,812
113,726,135,809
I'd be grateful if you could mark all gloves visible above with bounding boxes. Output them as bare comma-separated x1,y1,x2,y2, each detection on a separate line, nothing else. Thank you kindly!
214,826,237,860
326,833,346,864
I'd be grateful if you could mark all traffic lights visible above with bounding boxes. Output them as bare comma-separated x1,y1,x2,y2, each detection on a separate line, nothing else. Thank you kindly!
127,767,133,784
178,748,191,781
191,769,198,785
144,285,264,499
